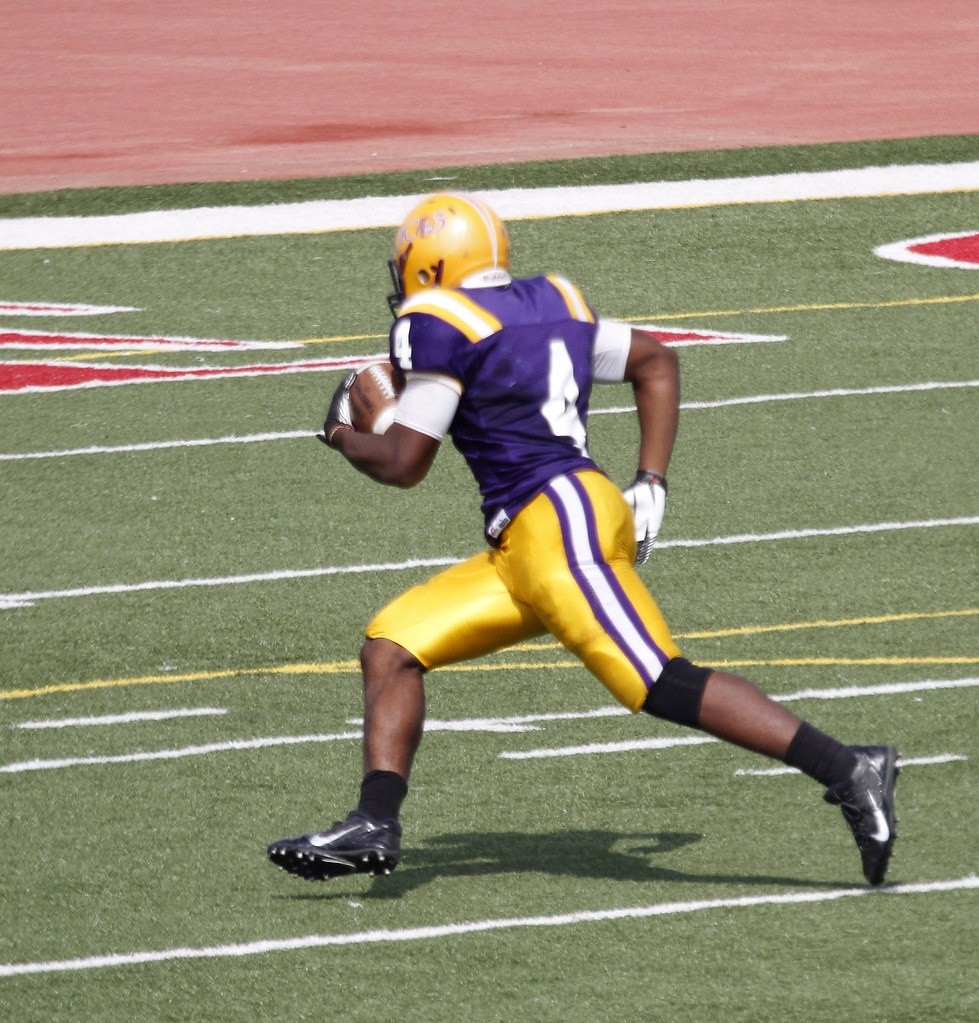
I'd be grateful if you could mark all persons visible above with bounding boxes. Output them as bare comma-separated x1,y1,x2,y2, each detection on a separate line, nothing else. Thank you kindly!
264,188,899,889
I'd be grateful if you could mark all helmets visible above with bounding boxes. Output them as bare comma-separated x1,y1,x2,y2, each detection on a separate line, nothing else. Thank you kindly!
392,190,511,298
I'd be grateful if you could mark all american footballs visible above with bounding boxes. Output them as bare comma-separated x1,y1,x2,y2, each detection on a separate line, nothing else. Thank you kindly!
349,358,406,435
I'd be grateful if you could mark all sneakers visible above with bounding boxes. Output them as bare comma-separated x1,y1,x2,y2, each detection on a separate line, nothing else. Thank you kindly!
267,811,404,881
823,743,904,887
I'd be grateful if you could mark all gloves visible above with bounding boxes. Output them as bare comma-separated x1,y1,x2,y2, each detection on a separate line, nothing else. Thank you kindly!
316,370,359,449
622,470,668,565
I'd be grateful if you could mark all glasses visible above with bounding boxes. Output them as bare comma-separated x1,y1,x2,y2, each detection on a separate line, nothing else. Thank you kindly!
387,258,405,320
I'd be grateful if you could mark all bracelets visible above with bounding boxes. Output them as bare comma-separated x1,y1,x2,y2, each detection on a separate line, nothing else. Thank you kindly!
329,426,353,449
